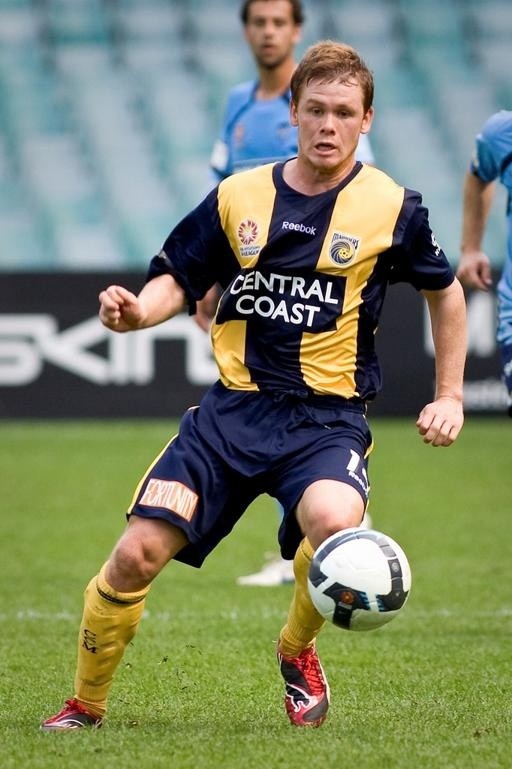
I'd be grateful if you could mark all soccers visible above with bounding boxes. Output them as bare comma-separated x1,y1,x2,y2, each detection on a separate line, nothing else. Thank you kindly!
307,527,411,632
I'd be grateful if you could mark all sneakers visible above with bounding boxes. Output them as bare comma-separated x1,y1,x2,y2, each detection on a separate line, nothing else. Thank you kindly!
39,697,102,731
276,637,331,729
236,558,295,587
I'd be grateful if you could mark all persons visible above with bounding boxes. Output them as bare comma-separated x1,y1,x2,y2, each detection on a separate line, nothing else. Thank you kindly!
37,40,468,735
191,0,376,587
456,106,512,419
